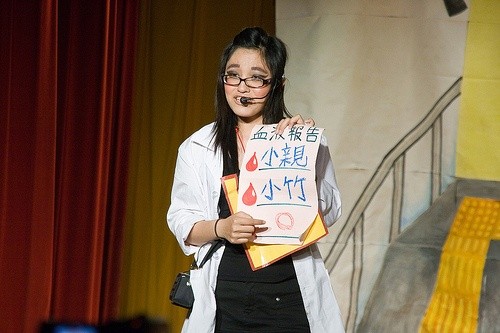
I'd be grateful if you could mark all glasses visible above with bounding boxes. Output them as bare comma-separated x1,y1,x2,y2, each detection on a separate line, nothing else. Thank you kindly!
221,75,271,88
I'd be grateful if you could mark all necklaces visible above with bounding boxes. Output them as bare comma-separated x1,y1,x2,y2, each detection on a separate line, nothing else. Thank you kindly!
236,129,245,155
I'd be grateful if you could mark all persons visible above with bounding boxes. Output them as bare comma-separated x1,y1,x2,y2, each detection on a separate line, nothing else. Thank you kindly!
168,27,341,333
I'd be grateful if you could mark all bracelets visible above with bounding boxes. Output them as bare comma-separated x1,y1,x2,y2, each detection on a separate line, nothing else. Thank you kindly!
214,219,220,238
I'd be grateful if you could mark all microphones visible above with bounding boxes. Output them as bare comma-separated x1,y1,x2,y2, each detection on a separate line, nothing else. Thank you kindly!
240,87,273,104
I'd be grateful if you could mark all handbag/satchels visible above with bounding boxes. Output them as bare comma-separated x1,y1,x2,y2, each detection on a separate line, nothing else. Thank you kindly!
169,272,194,308
